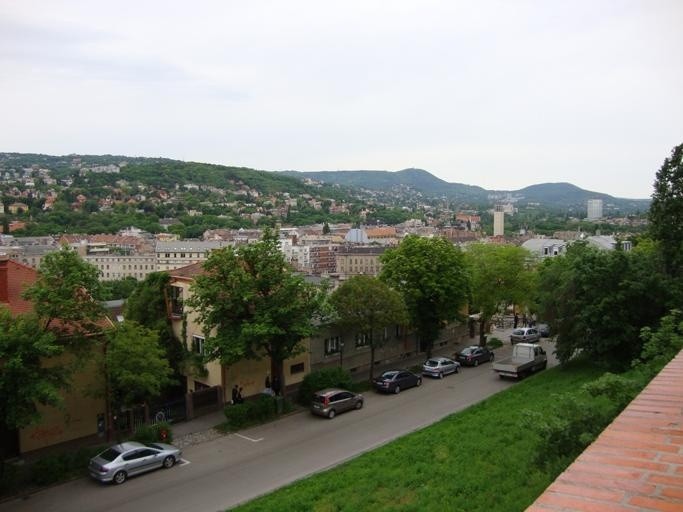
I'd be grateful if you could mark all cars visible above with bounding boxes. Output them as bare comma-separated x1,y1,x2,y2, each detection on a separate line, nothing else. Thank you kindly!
85,437,183,488
456,344,496,368
508,327,541,347
304,386,366,420
421,356,463,380
371,367,424,395
536,323,550,338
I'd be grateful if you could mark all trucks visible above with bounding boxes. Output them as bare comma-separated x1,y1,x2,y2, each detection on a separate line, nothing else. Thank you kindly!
492,342,548,383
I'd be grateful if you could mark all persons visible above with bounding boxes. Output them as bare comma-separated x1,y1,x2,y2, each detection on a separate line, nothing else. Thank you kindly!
271,376,281,397
231,384,239,405
467,318,478,339
513,311,537,329
264,375,271,394
235,387,243,404
